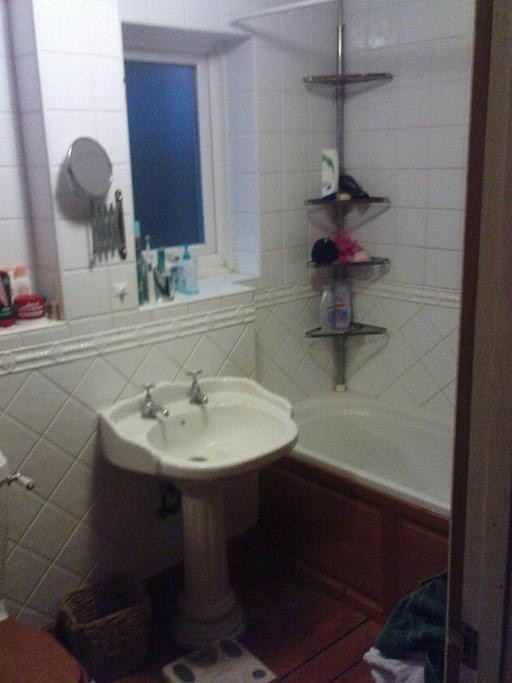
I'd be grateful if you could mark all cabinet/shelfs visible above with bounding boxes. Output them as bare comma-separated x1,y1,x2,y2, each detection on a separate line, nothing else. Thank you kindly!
300,0,394,393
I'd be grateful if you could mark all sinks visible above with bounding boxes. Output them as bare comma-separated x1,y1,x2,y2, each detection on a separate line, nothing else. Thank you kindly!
100,377,298,480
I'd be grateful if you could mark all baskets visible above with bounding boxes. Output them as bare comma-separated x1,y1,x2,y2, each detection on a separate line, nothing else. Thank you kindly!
60,569,152,682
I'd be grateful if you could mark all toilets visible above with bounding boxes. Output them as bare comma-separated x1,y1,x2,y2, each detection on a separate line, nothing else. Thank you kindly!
0,454,101,682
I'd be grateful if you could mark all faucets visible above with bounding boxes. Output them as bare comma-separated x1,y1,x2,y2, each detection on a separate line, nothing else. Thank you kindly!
187,370,208,404
142,382,170,418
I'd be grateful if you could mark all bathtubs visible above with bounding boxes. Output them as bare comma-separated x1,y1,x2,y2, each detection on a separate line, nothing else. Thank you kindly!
290,391,455,520
